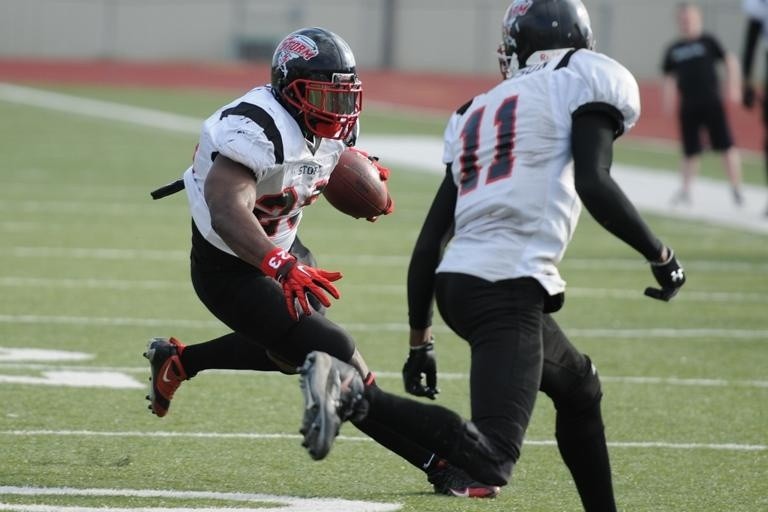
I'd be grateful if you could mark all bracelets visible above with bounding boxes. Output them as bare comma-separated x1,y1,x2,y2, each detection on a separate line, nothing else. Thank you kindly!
258,247,294,280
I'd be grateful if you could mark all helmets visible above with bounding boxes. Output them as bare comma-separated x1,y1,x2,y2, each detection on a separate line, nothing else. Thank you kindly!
496,0,594,77
272,27,363,140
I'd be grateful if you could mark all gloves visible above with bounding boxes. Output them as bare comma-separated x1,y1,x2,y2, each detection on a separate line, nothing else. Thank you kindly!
346,148,393,221
401,337,441,400
257,248,341,320
643,245,687,303
742,85,761,110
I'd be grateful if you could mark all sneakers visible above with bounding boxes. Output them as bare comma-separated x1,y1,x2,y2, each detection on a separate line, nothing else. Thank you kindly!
296,349,365,461
141,336,196,417
424,455,500,499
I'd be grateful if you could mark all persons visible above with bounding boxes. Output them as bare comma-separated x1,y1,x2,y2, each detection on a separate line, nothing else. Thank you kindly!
660,0,745,206
296,1,686,511
141,27,501,498
740,0,768,214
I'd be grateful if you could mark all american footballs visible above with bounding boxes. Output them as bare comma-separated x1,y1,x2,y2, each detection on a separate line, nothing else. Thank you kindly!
321,151,389,219
